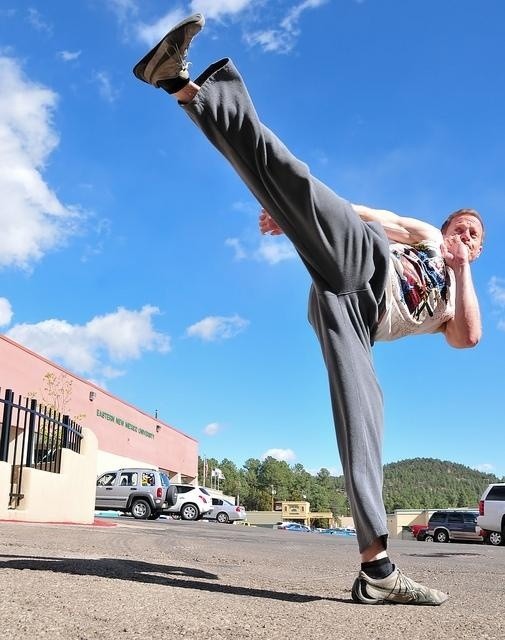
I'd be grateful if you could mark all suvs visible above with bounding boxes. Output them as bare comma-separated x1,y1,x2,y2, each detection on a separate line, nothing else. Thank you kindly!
475,482,505,546
125,483,214,520
426,510,503,546
94,467,178,520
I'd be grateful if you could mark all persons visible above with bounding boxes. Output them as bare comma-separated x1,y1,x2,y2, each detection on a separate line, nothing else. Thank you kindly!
132,12,484,607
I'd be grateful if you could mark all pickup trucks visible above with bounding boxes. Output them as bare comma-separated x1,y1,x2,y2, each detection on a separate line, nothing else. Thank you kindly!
412,524,429,537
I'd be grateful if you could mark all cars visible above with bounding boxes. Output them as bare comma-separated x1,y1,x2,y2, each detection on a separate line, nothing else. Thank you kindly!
273,521,357,536
202,495,247,524
416,528,434,542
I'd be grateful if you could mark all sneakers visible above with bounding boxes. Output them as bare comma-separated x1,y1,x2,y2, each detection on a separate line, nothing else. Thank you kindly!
132,13,205,89
352,563,449,606
